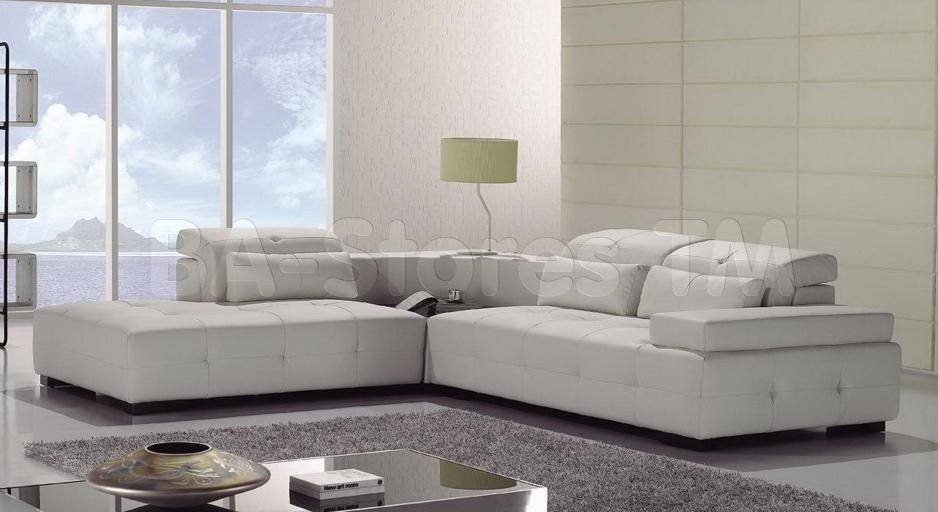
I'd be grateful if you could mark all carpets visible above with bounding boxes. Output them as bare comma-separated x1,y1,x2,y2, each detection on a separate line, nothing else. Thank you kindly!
24,407,903,512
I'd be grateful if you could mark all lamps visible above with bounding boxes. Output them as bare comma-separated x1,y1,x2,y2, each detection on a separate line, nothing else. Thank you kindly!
439,136,518,255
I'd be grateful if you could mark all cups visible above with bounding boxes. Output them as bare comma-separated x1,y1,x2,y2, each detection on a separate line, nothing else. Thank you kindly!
448,288,462,301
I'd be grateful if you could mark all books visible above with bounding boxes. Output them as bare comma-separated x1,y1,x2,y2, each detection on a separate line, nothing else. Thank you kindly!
289,490,386,511
289,468,386,501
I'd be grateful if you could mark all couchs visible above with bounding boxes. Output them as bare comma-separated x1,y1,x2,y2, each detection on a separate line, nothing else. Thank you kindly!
424,227,903,445
33,228,425,417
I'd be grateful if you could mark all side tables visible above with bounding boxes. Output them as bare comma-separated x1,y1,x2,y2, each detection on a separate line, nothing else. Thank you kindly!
434,294,485,314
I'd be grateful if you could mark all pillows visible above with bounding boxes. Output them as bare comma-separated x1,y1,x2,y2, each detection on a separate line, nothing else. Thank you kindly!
536,255,649,316
638,264,765,319
226,251,359,303
